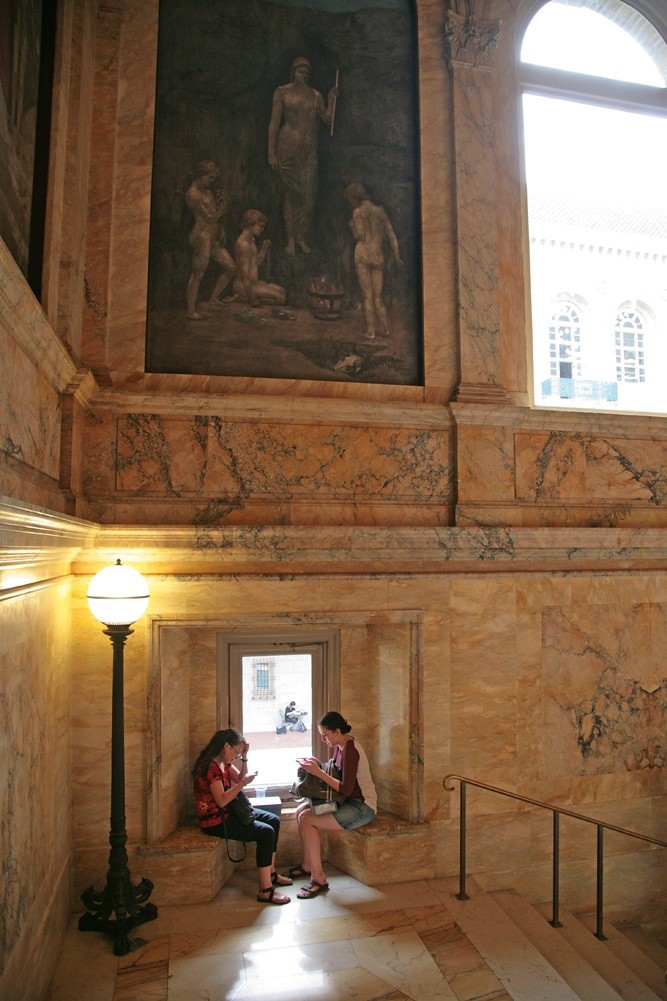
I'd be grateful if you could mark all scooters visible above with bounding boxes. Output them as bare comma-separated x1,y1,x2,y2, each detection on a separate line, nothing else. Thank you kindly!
276,711,308,735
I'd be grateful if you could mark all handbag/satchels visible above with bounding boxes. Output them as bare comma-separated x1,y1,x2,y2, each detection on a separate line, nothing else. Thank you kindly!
296,759,346,804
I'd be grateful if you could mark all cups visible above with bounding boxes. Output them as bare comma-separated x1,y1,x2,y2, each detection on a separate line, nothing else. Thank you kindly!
255,786,267,800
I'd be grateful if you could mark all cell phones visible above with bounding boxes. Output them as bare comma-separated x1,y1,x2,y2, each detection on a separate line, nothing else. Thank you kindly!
254,771,258,774
296,760,302,765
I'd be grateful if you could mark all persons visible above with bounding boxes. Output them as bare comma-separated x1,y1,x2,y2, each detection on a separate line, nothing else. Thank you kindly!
281,711,378,899
285,701,298,724
191,727,293,905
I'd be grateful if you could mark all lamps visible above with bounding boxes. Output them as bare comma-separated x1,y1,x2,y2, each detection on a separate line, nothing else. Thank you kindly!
86,557,150,626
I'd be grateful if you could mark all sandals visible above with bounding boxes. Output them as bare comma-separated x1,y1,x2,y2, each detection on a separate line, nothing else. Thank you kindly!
257,887,290,904
271,872,292,885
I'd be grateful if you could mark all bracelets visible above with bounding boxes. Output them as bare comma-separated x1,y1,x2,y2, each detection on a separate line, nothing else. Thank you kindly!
242,780,246,786
241,759,248,762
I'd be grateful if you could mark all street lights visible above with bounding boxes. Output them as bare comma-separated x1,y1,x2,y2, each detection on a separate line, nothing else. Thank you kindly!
79,558,168,952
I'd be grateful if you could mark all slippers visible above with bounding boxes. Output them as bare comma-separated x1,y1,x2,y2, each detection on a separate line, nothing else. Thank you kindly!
296,879,329,899
282,866,310,878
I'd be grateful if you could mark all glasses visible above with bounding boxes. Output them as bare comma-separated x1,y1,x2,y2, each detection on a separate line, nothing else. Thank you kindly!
230,743,243,759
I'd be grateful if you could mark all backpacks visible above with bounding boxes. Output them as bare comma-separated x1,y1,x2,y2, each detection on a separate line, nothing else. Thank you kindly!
218,786,256,862
